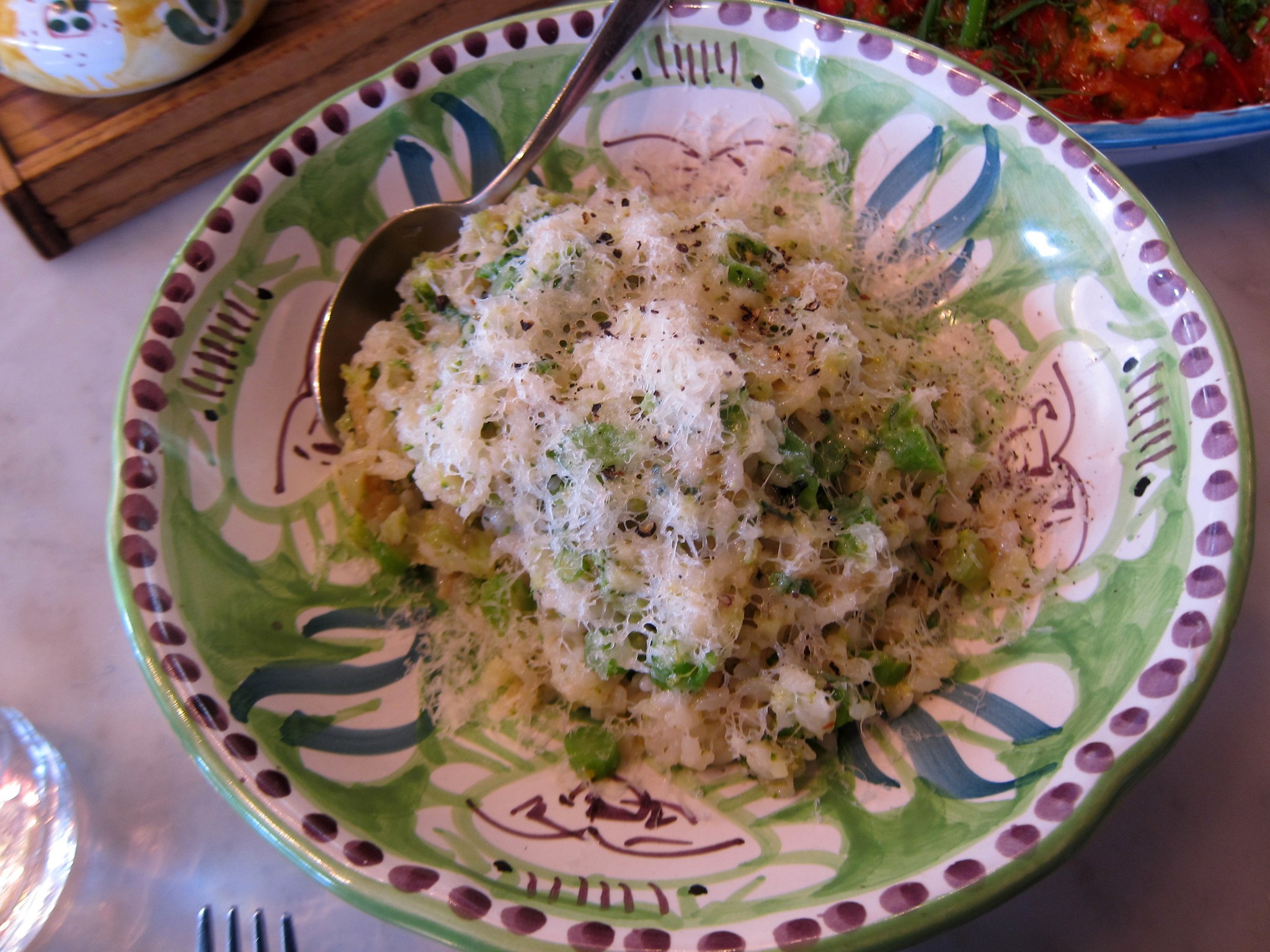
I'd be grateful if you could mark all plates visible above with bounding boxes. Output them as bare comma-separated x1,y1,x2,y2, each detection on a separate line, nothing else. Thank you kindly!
107,0,1257,952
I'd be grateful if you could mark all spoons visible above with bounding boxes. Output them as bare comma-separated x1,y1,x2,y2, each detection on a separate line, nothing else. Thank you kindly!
311,0,666,450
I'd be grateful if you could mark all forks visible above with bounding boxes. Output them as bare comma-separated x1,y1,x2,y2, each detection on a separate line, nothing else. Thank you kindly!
196,906,298,952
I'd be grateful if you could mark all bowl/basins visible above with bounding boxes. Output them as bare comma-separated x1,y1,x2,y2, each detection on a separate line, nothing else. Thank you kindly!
1067,105,1270,166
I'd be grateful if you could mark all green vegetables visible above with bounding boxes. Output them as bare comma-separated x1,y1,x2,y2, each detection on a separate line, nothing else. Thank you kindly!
803,0,1270,107
379,224,988,777
